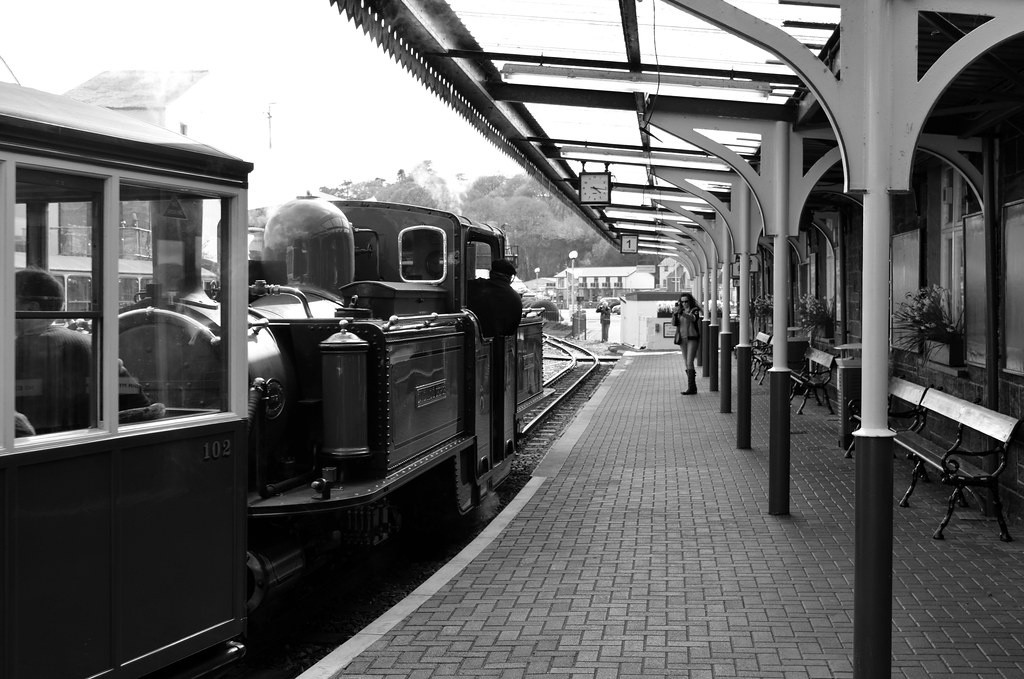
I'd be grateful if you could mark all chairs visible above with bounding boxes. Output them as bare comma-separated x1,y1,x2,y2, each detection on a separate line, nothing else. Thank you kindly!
790,347,842,415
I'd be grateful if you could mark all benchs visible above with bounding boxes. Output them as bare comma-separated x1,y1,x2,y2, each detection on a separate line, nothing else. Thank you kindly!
754,337,774,385
844,374,935,481
750,331,771,376
894,387,1022,542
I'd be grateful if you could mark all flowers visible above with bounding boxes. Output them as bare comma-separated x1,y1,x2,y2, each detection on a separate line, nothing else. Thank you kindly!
796,294,835,343
889,284,963,368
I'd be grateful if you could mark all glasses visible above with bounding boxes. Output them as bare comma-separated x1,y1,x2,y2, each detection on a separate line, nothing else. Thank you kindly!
680,300,689,303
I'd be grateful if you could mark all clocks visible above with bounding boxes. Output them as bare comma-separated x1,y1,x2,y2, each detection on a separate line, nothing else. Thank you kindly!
579,172,610,206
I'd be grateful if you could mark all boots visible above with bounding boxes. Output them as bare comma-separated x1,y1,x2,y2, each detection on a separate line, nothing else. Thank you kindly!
681,369,697,395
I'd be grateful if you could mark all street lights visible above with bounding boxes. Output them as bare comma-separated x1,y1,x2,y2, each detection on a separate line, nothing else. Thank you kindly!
568,249,578,321
533,265,540,295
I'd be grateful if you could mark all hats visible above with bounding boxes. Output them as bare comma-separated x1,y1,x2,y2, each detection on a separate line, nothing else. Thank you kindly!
491,260,517,276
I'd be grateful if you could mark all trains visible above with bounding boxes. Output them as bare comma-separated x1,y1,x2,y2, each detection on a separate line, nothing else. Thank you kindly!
1,82,545,679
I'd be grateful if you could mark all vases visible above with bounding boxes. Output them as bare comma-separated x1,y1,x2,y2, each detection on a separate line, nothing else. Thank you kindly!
925,339,964,366
811,323,834,338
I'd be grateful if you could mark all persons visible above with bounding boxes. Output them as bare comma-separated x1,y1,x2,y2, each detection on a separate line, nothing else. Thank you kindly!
15,270,149,434
596,301,611,343
671,293,704,394
475,259,522,338
